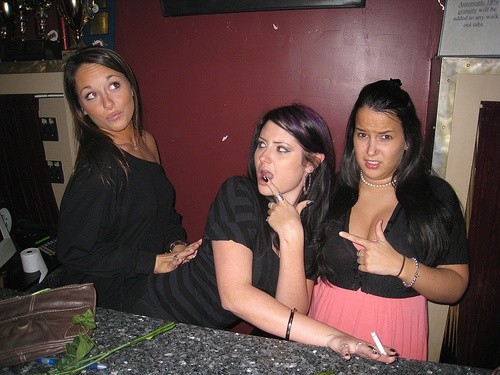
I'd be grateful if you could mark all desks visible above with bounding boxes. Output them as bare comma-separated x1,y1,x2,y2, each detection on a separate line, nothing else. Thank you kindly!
0,285,500,375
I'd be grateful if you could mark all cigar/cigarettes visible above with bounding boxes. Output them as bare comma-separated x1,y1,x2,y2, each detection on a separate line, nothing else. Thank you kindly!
372,332,389,364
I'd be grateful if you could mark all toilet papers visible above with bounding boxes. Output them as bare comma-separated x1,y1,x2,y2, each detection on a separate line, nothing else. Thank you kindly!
19,248,49,284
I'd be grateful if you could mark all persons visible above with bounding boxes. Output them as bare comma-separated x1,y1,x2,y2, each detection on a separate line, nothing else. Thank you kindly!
55,46,202,308
126,102,396,363
307,79,469,361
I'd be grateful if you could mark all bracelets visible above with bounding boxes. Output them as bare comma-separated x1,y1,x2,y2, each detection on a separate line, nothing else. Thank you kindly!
170,242,188,252
286,308,297,341
403,258,419,287
395,255,405,277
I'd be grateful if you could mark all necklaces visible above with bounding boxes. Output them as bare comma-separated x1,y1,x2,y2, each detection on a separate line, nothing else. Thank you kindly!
360,170,396,187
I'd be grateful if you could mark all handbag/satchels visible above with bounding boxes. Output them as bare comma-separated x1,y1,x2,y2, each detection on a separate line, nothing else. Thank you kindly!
0,282,96,366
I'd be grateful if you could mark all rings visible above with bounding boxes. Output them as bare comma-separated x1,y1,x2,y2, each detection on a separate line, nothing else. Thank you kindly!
356,342,363,354
274,193,285,203
174,254,179,260
358,250,361,257
